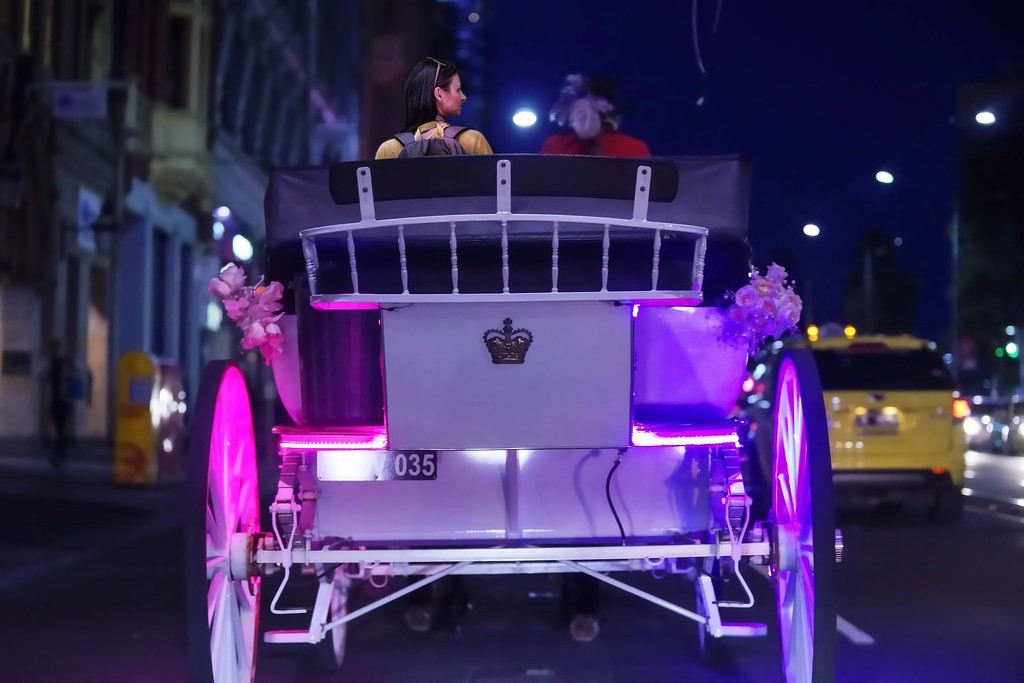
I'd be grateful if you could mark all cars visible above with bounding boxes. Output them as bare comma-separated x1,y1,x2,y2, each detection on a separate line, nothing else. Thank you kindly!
804,330,970,517
967,391,1023,461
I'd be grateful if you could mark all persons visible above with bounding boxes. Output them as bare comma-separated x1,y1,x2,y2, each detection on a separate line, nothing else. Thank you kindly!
375,56,493,161
542,73,649,158
38,339,77,463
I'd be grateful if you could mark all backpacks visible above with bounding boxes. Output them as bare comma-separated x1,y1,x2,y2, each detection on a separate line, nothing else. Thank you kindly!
393,125,470,156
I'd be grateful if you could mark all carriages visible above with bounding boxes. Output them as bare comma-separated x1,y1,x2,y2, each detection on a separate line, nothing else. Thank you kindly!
181,141,831,682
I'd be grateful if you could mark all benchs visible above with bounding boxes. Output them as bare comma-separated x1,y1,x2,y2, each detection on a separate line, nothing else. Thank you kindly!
263,154,748,305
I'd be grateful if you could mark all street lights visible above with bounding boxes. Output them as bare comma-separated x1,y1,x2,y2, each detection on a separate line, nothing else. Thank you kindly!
950,104,1004,396
798,217,824,321
860,163,896,330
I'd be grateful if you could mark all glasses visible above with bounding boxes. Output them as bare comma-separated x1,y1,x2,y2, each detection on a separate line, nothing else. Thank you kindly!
426,54,447,88
